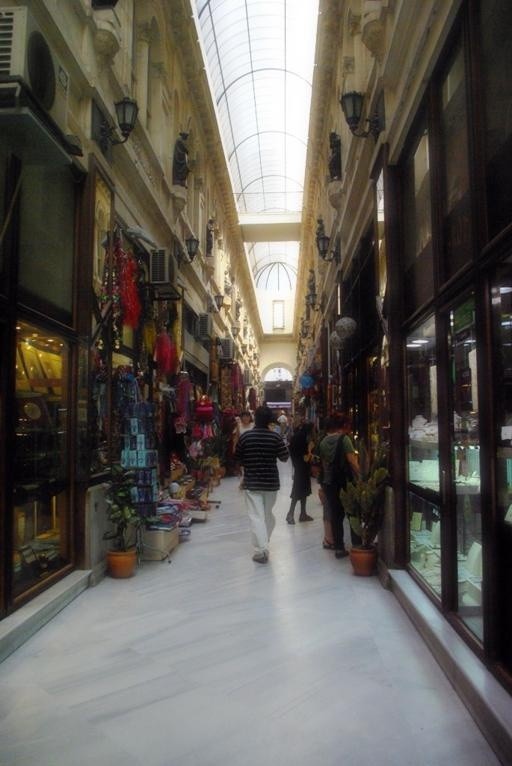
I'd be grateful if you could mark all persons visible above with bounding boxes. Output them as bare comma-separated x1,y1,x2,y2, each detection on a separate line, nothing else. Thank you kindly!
232,415,242,453
269,410,362,560
235,410,254,484
236,404,290,564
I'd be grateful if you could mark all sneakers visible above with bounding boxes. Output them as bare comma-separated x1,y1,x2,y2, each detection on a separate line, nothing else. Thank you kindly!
322,541,336,549
254,555,267,563
300,514,313,521
335,548,349,558
286,515,295,524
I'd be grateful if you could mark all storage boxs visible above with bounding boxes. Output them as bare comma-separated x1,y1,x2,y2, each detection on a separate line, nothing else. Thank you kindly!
123,523,179,561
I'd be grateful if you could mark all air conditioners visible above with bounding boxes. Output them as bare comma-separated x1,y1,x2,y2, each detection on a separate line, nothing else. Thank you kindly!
149,248,179,294
0,6,69,134
243,369,253,385
221,338,235,361
197,312,213,341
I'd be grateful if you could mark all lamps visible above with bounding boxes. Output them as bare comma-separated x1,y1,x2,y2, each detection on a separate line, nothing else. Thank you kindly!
206,292,224,313
338,90,380,138
176,235,199,267
316,231,341,265
300,324,315,341
306,290,323,312
100,97,139,153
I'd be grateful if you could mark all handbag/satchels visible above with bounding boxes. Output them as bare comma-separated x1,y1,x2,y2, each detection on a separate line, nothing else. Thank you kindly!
331,456,355,490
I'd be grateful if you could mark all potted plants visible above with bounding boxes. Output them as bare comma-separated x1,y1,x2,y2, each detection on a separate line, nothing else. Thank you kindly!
102,465,146,578
340,438,391,575
